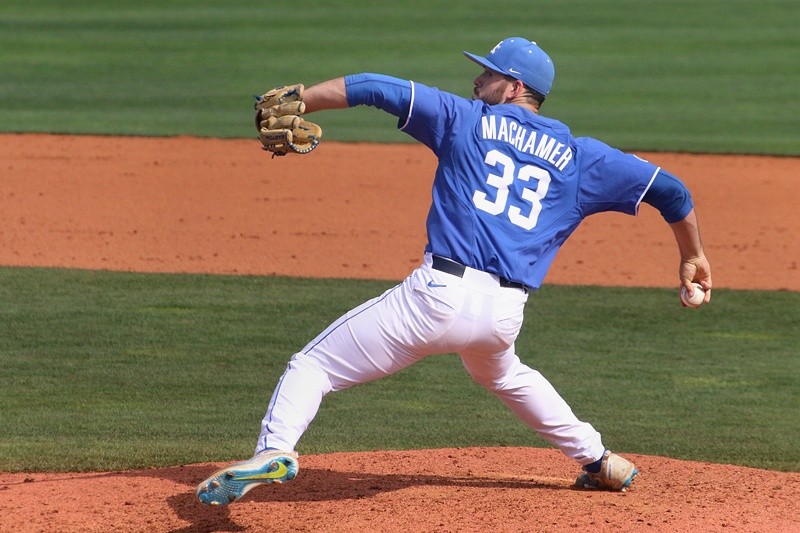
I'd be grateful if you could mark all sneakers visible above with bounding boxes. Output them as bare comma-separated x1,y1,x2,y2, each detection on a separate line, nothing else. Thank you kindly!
576,448,638,492
195,449,299,506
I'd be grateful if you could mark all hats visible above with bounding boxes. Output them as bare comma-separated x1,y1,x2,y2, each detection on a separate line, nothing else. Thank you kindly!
464,36,555,96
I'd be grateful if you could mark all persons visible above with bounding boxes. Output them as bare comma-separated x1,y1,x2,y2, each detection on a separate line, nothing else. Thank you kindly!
197,37,713,505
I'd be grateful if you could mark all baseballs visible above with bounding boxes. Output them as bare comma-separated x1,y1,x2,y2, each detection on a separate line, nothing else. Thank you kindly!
681,282,706,309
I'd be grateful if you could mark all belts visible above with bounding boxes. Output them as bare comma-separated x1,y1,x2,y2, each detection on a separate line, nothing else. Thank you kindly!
431,256,522,288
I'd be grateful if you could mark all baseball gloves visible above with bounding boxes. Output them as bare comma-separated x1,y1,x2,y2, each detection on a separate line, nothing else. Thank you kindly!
254,82,322,158
